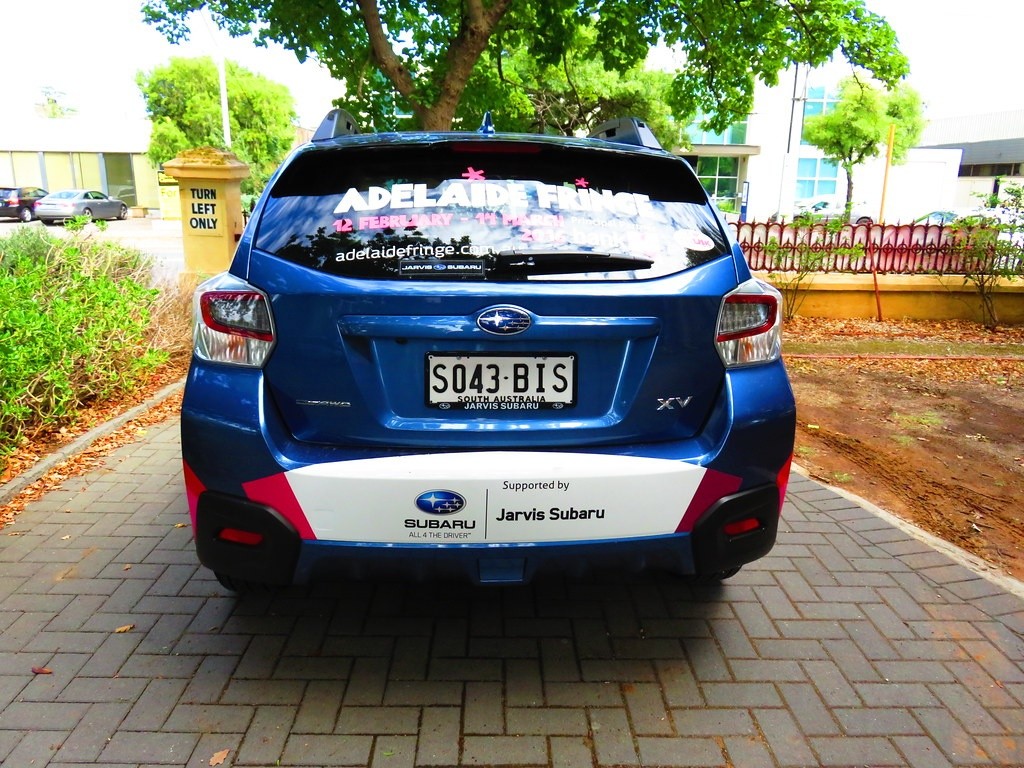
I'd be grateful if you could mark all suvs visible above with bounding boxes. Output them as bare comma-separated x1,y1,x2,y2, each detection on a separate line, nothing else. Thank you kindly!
180,109,800,619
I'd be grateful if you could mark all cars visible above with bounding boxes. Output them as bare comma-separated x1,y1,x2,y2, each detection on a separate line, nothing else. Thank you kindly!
33,189,129,228
793,194,877,226
910,207,1024,248
0,186,50,222
